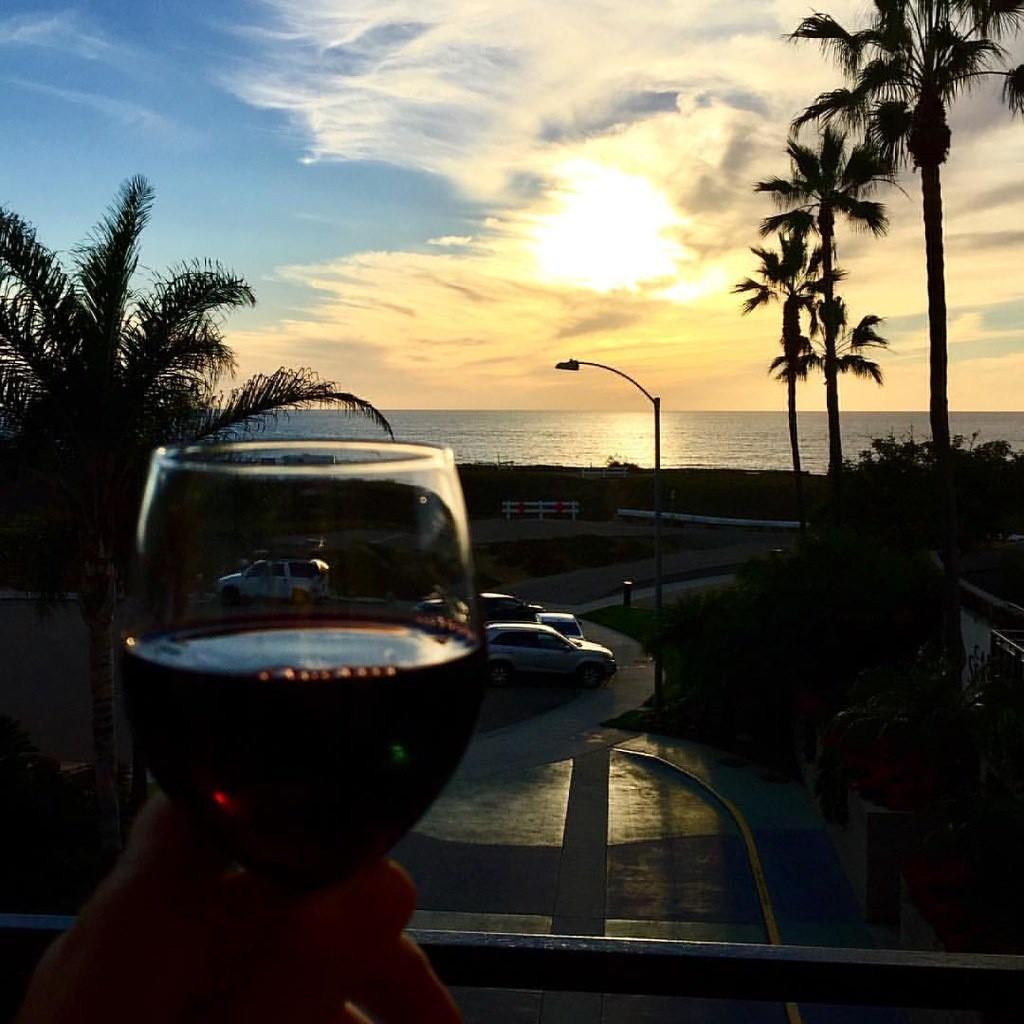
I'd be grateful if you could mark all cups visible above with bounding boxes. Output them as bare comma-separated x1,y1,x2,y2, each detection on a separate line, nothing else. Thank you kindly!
118,437,488,893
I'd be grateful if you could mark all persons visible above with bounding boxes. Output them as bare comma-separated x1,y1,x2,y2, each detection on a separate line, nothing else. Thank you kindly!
19,790,464,1024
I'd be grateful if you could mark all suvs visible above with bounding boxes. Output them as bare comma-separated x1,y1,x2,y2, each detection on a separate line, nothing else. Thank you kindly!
217,559,331,608
485,623,618,688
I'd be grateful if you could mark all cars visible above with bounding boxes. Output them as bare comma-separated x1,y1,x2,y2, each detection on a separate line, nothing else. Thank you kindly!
532,612,584,640
413,593,547,623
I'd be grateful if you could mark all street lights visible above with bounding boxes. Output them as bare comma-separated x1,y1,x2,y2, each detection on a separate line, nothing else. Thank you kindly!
555,358,663,609
